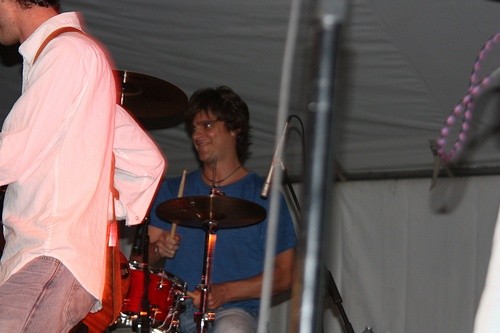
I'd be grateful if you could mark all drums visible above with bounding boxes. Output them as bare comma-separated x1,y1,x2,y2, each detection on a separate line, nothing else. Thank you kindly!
120,259,180,330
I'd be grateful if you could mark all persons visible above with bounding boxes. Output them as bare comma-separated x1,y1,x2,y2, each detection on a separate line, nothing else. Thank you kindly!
0,0,166,333
130,84,300,333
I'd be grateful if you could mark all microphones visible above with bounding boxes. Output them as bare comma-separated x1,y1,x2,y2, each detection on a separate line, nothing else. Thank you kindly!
260,115,293,200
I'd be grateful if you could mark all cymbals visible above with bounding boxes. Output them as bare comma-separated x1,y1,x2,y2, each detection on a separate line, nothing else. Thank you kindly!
157,193,268,230
114,68,190,131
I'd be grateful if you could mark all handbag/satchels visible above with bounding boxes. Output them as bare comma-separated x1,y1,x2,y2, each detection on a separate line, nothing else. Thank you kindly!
79,246,134,333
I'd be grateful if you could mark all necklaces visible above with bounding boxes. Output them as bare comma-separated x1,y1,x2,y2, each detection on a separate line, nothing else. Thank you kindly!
202,165,242,183
